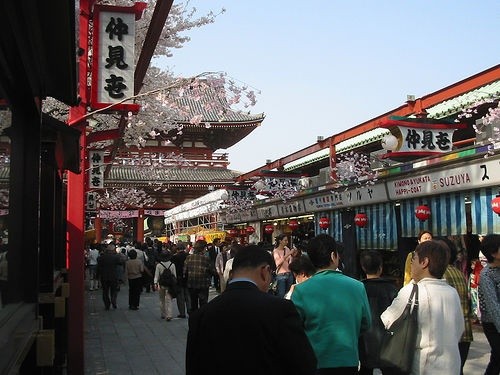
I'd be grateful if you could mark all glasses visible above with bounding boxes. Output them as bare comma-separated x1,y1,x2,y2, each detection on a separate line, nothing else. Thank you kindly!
261,265,276,281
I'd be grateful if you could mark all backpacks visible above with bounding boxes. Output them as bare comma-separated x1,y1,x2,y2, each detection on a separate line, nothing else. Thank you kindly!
158,262,177,288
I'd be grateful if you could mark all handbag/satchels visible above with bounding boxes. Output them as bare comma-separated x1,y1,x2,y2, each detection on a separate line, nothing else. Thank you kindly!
377,282,419,375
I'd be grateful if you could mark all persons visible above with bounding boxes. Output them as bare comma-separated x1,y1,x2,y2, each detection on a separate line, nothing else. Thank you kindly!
85,232,314,322
284,230,500,375
185,244,318,375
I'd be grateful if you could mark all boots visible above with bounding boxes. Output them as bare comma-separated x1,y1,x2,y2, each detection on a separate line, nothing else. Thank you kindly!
89,279,100,290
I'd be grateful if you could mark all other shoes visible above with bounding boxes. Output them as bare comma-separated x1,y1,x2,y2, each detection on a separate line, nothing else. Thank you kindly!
161,316,165,319
129,306,139,310
146,290,150,293
112,304,117,309
105,306,110,310
166,317,172,321
177,314,186,318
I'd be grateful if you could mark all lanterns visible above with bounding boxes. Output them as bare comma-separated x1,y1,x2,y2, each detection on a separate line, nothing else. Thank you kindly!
255,181,264,190
221,193,229,201
289,220,299,231
414,204,432,223
230,226,255,238
319,217,331,230
381,135,398,150
491,194,500,217
354,212,368,228
265,224,274,234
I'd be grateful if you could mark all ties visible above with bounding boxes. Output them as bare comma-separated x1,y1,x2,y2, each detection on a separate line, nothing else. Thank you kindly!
217,248,218,254
